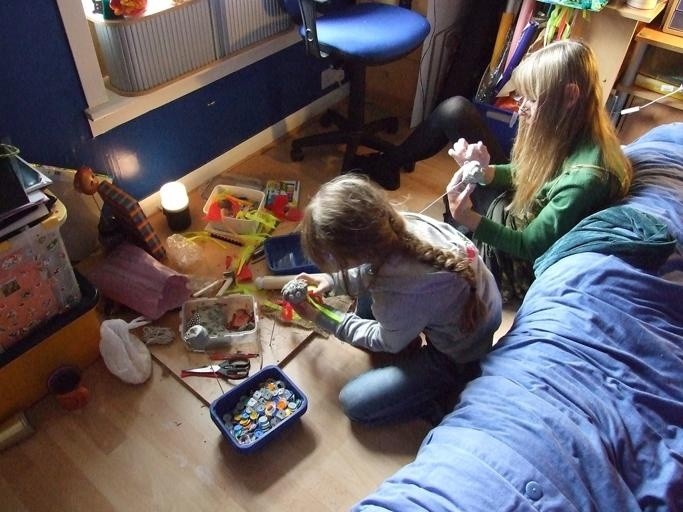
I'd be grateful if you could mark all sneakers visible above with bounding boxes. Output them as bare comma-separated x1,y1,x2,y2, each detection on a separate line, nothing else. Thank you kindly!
344,148,402,192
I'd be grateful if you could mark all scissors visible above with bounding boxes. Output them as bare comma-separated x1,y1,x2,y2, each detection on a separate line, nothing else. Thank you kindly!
181,357,250,379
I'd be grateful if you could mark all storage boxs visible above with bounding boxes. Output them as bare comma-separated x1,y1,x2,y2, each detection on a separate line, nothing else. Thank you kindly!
0,187,107,423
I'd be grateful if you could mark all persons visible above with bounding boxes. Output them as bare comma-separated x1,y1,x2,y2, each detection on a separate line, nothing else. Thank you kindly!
347,38,637,298
281,173,508,424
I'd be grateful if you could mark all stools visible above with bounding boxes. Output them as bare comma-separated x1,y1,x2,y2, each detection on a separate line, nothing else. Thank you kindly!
284,1,429,178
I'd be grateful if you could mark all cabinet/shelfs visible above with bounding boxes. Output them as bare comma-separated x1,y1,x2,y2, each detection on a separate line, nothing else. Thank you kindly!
617,27,683,112
